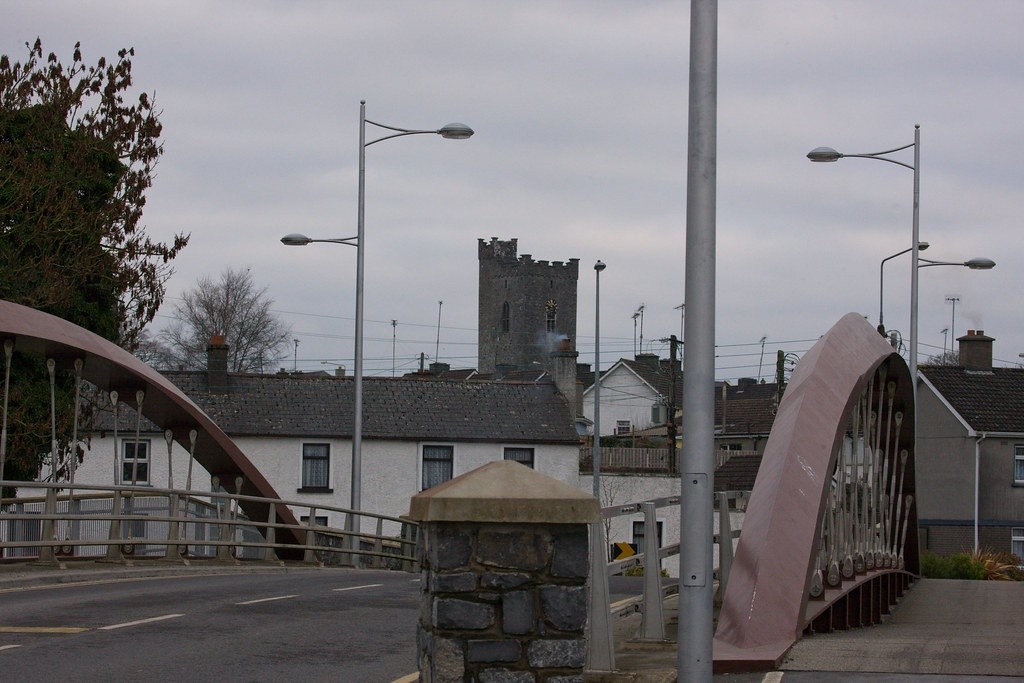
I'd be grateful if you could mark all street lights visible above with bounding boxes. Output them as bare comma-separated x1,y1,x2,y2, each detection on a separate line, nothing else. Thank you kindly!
879,241,930,338
806,123,995,404
279,100,474,570
591,259,606,495
